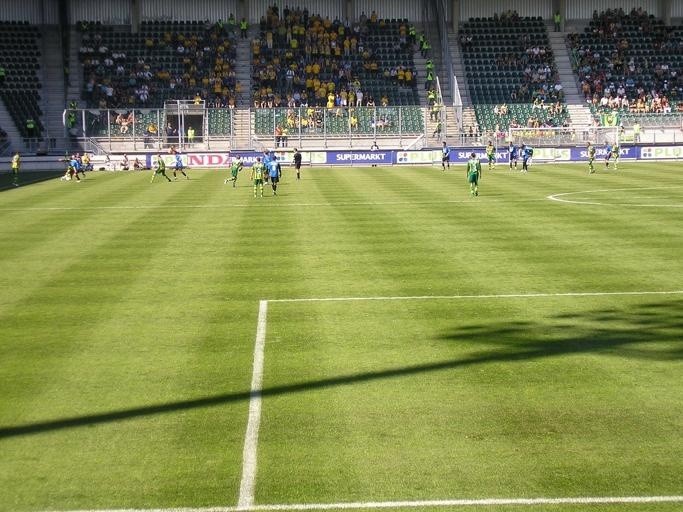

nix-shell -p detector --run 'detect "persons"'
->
[249,157,266,198]
[10,151,21,187]
[467,151,483,197]
[568,6,682,117]
[224,154,243,186]
[78,12,248,149]
[0,65,5,83]
[265,156,281,197]
[595,119,641,142]
[25,114,36,138]
[61,152,189,183]
[441,142,450,170]
[252,4,441,137]
[458,8,575,143]
[294,148,301,180]
[275,124,288,148]
[587,141,620,173]
[486,141,531,171]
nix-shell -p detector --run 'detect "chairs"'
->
[72,8,683,139]
[0,18,44,148]
